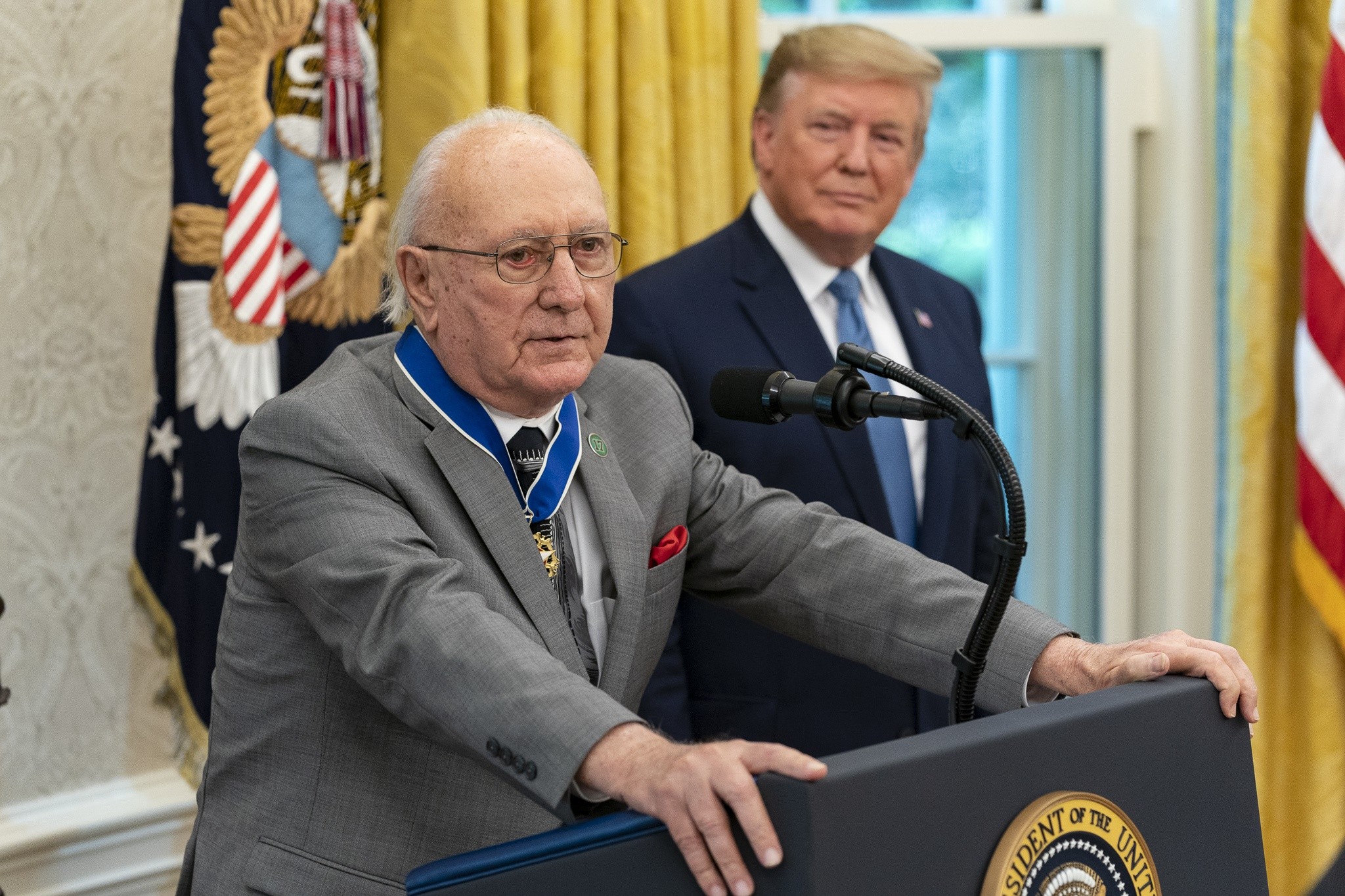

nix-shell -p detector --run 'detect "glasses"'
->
[417,230,628,284]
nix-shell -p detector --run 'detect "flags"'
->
[1291,2,1345,659]
[126,2,393,797]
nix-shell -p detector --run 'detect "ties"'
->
[828,269,919,550]
[505,426,600,688]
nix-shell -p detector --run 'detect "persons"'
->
[177,106,1260,896]
[602,28,1011,759]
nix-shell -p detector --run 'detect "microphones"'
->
[710,365,945,431]
